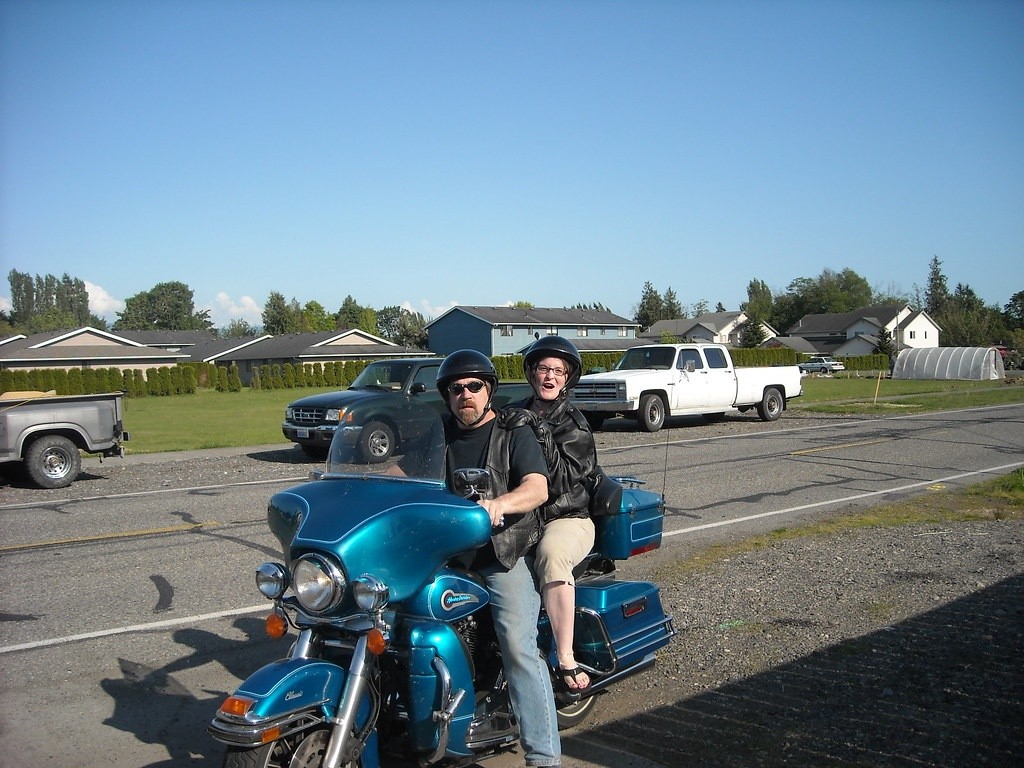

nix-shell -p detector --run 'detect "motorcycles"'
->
[207,393,679,768]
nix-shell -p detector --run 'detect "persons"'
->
[385,349,566,768]
[498,334,597,695]
[889,351,897,376]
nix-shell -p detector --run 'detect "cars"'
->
[795,356,846,374]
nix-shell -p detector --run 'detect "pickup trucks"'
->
[566,343,804,433]
[281,356,541,466]
[0,389,131,488]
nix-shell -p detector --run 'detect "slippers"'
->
[555,661,591,693]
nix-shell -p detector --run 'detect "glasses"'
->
[446,380,487,396]
[535,365,567,375]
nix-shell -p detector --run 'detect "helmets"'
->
[523,336,582,398]
[436,349,498,396]
